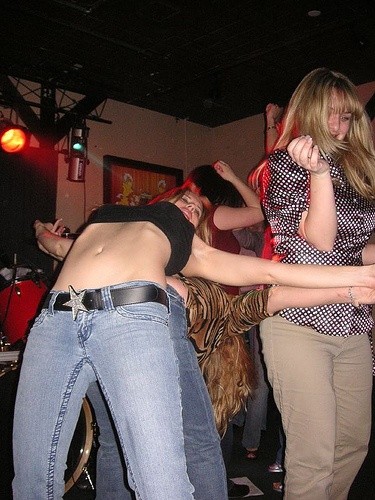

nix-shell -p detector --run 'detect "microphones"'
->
[66,151,86,183]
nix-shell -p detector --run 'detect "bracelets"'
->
[264,126,276,130]
[348,287,358,307]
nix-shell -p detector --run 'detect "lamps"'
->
[66,125,90,182]
[0,111,30,156]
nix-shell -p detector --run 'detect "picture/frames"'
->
[102,154,184,207]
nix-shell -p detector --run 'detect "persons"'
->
[11,188,375,500]
[34,96,375,499]
[81,274,375,500]
[257,67,375,500]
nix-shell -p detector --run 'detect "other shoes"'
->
[245,446,258,458]
[272,479,283,493]
[268,463,284,473]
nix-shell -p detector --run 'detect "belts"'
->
[42,284,169,313]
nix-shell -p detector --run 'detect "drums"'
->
[2,273,47,346]
[0,361,93,497]
